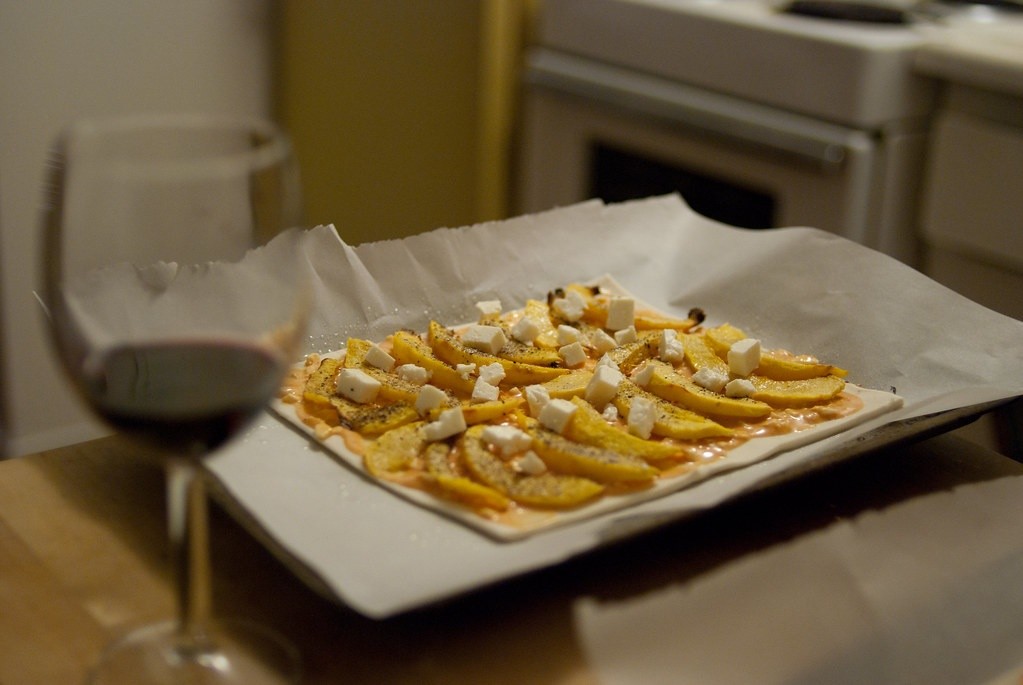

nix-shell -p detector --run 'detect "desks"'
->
[0,432,1023,685]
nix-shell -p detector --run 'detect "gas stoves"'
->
[532,0,1002,129]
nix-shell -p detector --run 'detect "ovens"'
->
[511,44,937,279]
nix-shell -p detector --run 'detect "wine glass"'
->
[41,114,310,685]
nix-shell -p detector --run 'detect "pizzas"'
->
[277,276,861,546]
